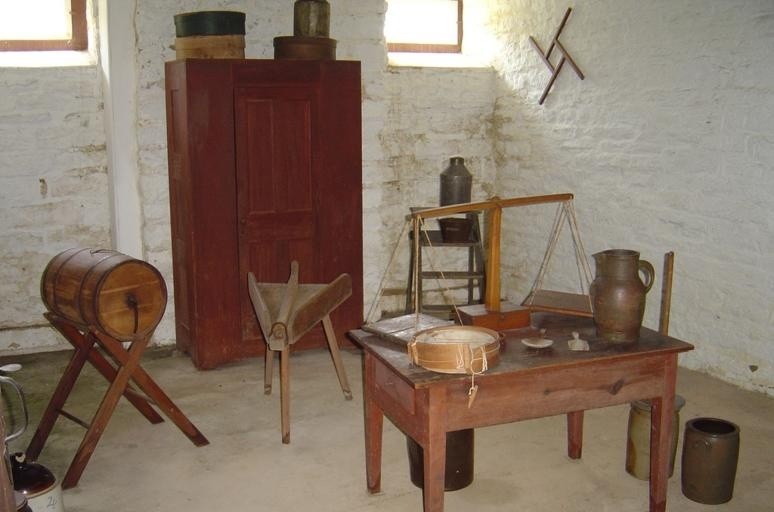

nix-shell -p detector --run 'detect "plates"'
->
[521,337,553,348]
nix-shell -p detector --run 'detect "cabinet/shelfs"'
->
[164,58,364,371]
[406,206,486,315]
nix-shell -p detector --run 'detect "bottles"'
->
[439,157,472,240]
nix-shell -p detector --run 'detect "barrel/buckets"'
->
[42,245,167,342]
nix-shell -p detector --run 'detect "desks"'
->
[345,311,695,512]
